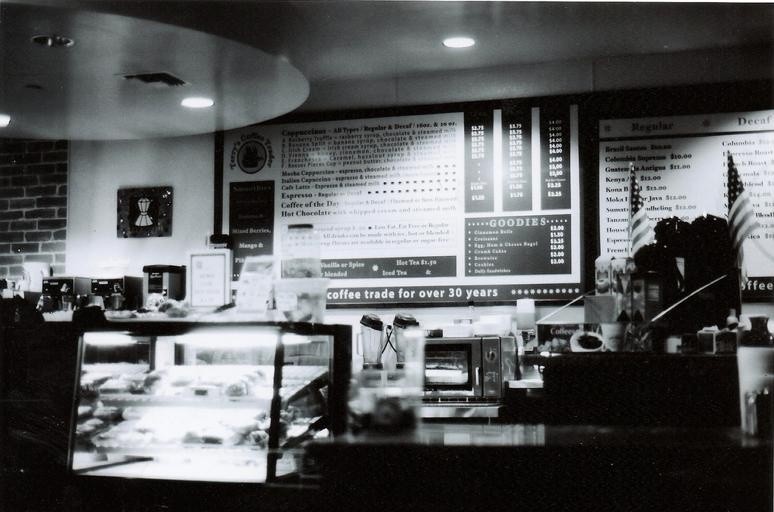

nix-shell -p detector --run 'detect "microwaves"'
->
[422,336,516,406]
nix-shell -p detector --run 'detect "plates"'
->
[569,332,602,352]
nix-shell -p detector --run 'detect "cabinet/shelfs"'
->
[61,318,352,510]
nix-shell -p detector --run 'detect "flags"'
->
[728,147,761,292]
[628,162,656,258]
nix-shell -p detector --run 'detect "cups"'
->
[599,323,624,351]
[516,298,536,330]
[424,315,513,339]
[354,314,382,370]
[270,276,331,323]
[385,313,419,368]
[666,338,681,353]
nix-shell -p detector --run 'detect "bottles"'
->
[733,314,773,437]
[280,223,325,277]
[727,308,738,332]
